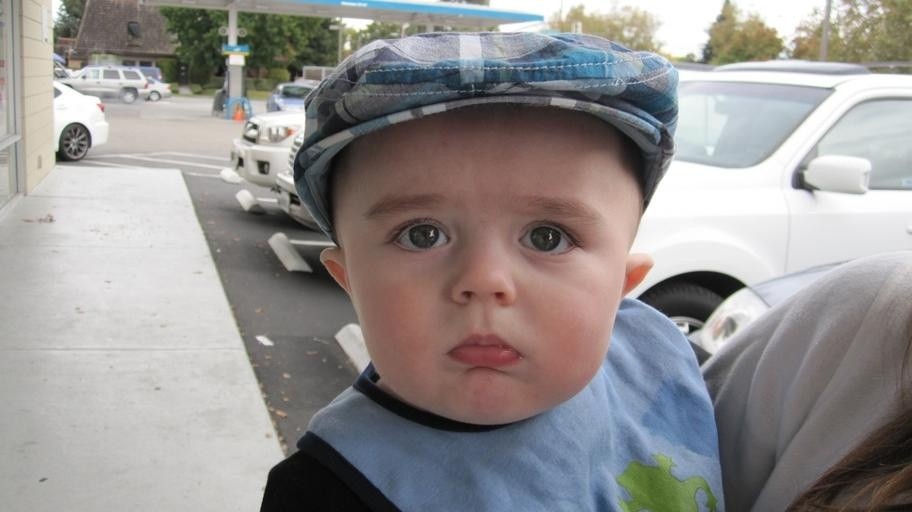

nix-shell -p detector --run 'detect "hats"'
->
[293,29,678,243]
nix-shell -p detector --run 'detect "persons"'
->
[702,234,912,512]
[259,31,725,512]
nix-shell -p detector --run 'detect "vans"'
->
[52,62,163,103]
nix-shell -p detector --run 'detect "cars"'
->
[53,79,110,160]
[141,76,172,103]
